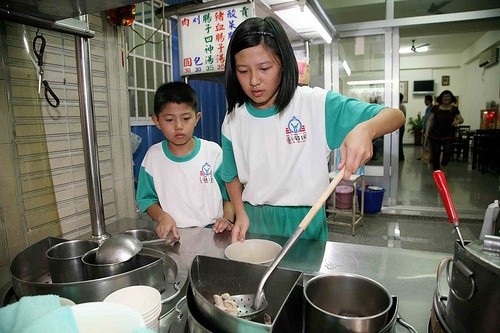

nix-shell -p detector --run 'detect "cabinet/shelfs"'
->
[480,109,498,129]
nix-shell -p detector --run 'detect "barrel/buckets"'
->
[446,241,500,333]
[335,186,353,209]
[357,185,385,215]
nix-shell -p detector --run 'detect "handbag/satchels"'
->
[421,139,430,164]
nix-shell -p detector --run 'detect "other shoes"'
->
[443,167,448,177]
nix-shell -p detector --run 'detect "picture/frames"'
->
[399,81,408,103]
[442,75,450,86]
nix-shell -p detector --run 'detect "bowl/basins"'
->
[82,247,137,279]
[102,286,163,333]
[57,297,75,308]
[304,274,392,333]
[225,239,283,264]
[45,240,100,283]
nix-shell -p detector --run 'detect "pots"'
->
[186,256,398,333]
[427,258,456,333]
[10,236,165,303]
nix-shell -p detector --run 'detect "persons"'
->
[222,18,405,244]
[369,90,464,174]
[136,81,234,239]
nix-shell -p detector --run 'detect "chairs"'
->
[456,125,470,158]
[324,148,365,237]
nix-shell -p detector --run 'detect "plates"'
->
[74,302,144,333]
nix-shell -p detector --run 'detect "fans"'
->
[411,40,430,53]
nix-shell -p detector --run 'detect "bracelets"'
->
[227,219,233,224]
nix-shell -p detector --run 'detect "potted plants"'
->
[406,115,425,146]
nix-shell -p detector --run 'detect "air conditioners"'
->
[478,47,500,68]
